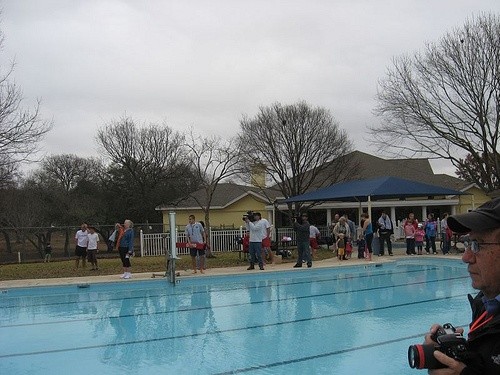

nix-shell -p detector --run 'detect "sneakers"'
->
[120,272,129,278]
[124,273,133,279]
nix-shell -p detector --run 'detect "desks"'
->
[438,233,466,255]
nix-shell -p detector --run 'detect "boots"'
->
[342,254,348,260]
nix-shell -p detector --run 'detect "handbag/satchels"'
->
[378,228,391,240]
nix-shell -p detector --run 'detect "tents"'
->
[276,176,475,203]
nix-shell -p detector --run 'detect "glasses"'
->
[464,240,500,254]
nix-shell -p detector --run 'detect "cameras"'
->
[408,329,467,369]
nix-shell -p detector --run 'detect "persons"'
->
[185,214,208,274]
[293,211,394,267]
[403,197,500,375]
[242,210,274,270]
[44,242,52,263]
[75,223,99,271]
[109,219,134,278]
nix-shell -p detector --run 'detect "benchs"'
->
[232,248,294,261]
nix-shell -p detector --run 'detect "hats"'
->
[447,198,500,234]
[338,216,346,222]
[418,223,422,228]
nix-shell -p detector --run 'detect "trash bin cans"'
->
[372,237,381,255]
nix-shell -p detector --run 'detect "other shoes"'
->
[260,265,265,270]
[307,262,312,267]
[378,253,381,256]
[433,252,437,255]
[427,252,430,255]
[411,253,414,256]
[389,252,393,256]
[405,252,408,256]
[246,265,254,270]
[293,262,302,267]
